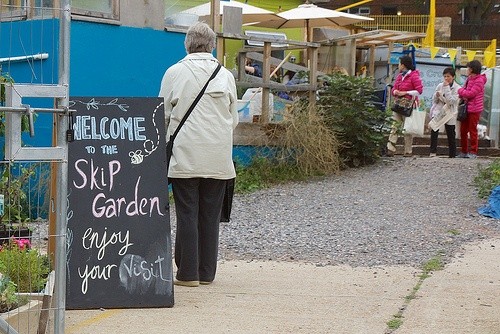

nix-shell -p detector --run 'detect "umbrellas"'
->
[165,0,288,67]
[243,0,375,67]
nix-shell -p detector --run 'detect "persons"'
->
[158,22,239,287]
[430,68,461,157]
[455,60,487,158]
[245,57,350,101]
[387,56,424,156]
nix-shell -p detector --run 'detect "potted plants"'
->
[0,276,43,334]
[0,165,37,244]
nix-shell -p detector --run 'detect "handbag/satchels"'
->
[457,100,467,121]
[166,140,173,185]
[391,96,413,117]
[402,101,426,137]
[220,162,236,223]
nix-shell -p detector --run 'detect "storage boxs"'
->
[237,100,254,124]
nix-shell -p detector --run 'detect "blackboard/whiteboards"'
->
[65,95,175,311]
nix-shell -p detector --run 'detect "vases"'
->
[14,270,54,334]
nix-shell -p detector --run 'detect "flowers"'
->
[0,236,51,293]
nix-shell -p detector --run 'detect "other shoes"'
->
[383,151,394,157]
[429,152,436,157]
[174,278,210,287]
[454,153,477,158]
[403,153,412,157]
